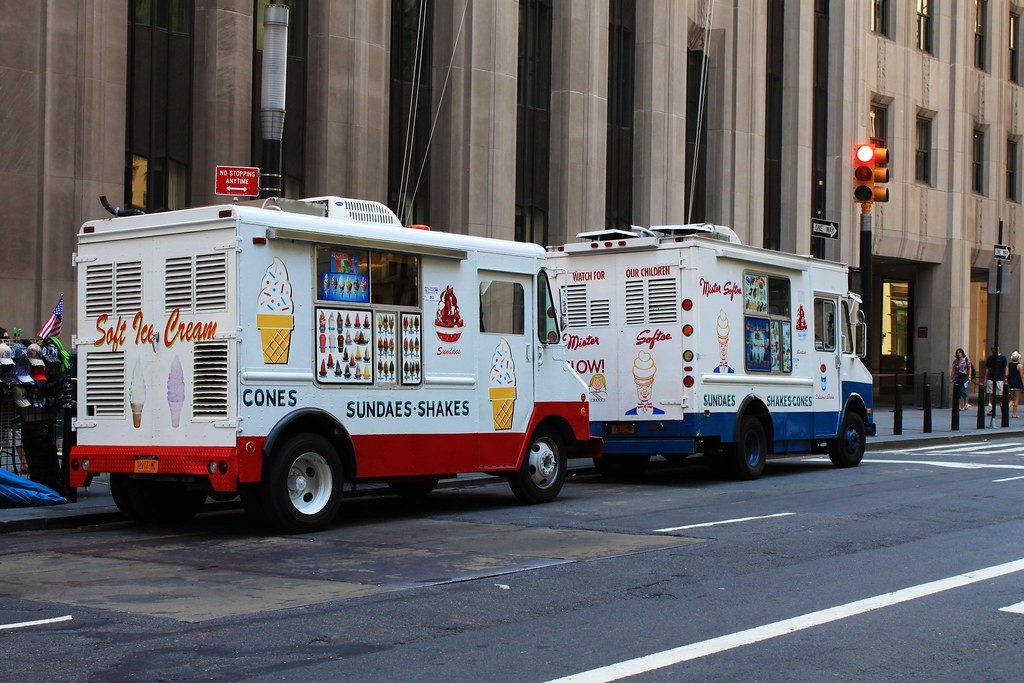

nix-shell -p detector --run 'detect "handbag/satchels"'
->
[966,358,976,380]
[957,372,967,385]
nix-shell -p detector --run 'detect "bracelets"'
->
[969,377,971,378]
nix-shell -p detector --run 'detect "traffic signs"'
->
[214,166,260,197]
[992,243,1012,262]
[810,217,839,240]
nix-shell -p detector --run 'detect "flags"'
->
[35,293,63,340]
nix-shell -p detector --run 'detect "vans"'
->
[544,223,877,483]
[69,195,603,535]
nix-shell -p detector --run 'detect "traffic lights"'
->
[852,144,875,201]
[874,146,890,202]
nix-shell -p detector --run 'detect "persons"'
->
[983,345,1008,416]
[950,348,972,412]
[0,328,30,473]
[1005,350,1024,418]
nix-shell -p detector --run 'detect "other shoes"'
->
[1012,415,1020,418]
[21,467,29,474]
[964,404,972,410]
[987,410,992,415]
[959,407,965,411]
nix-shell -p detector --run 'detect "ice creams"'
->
[324,274,367,299]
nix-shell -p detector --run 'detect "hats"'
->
[0,341,72,409]
[1011,351,1021,360]
